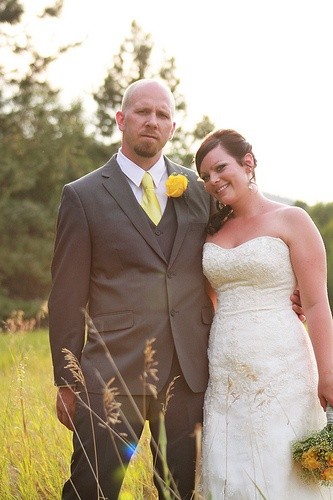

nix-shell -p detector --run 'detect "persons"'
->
[194,129,333,500]
[48,78,308,500]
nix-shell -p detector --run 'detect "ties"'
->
[140,172,161,226]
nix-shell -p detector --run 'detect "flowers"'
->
[292,403,333,489]
[164,172,201,221]
